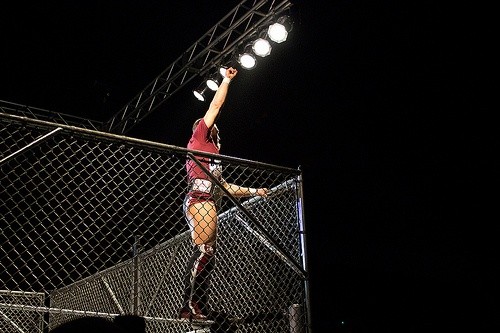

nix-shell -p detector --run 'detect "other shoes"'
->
[178,311,224,323]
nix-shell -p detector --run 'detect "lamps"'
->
[191,13,295,103]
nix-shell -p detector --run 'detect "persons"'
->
[182,66,268,321]
